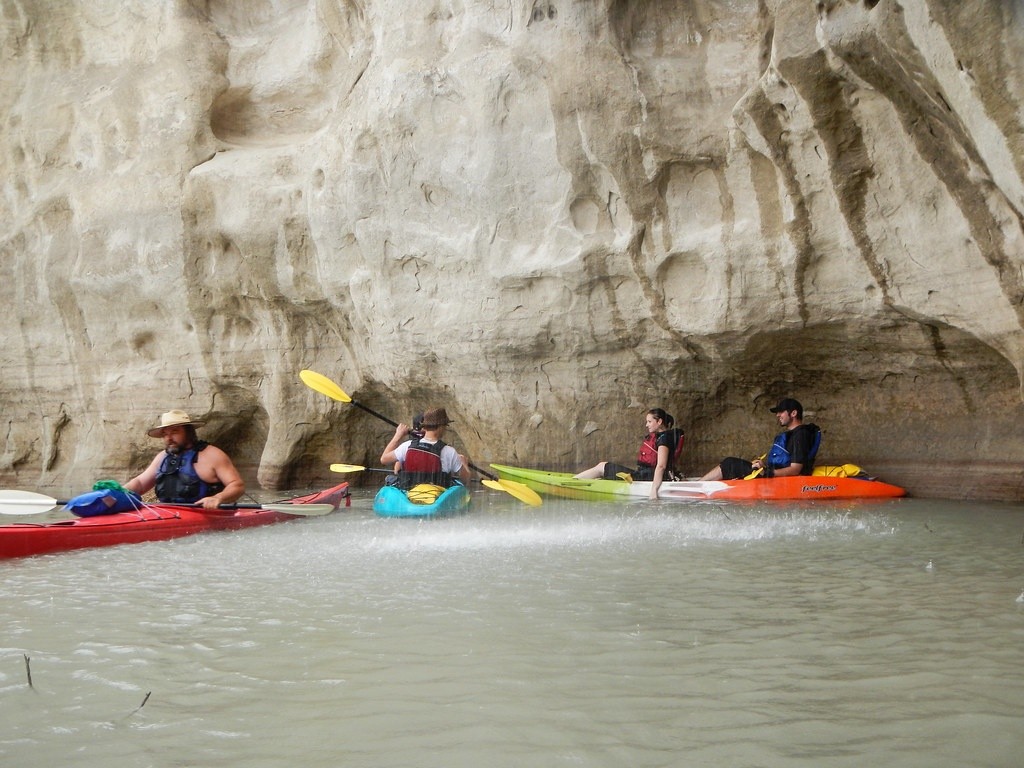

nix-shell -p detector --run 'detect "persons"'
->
[123,410,246,509]
[381,407,471,485]
[668,398,812,482]
[572,408,674,500]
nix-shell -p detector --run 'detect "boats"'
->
[489,459,907,501]
[368,478,474,521]
[0,479,351,560]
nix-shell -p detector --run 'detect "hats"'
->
[413,415,424,432]
[770,399,803,415]
[419,408,454,426]
[148,411,207,438]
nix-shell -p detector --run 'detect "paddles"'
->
[616,470,636,486]
[744,452,768,481]
[330,462,505,492]
[0,489,337,518]
[298,368,543,508]
